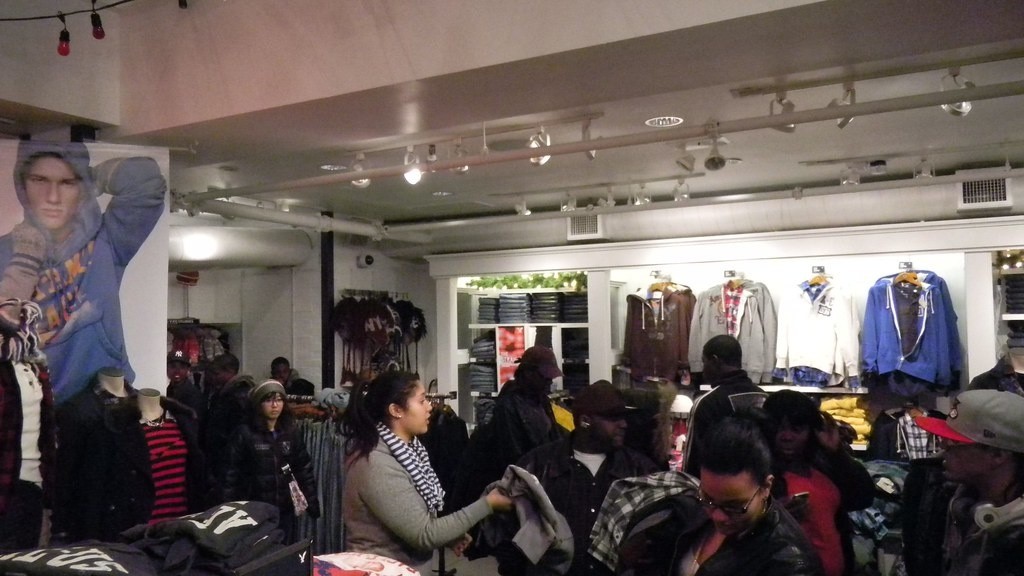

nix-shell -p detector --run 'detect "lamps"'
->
[769,80,854,132]
[677,126,725,171]
[349,153,371,188]
[525,114,602,166]
[941,69,977,117]
[791,153,943,198]
[517,177,690,216]
[403,121,490,184]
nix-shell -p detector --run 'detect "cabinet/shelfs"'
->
[467,287,589,397]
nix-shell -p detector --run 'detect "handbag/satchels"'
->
[285,472,309,517]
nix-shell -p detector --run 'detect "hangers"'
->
[894,263,921,287]
[807,266,827,286]
[168,318,202,333]
[549,389,573,407]
[646,271,675,302]
[722,270,746,290]
[425,392,454,418]
[286,395,337,423]
[672,410,691,438]
[903,398,924,420]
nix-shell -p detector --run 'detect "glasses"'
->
[936,437,985,455]
[694,477,765,519]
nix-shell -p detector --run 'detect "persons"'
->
[0,141,168,399]
[0,296,187,552]
[339,370,514,576]
[964,346,1024,399]
[483,336,1024,576]
[167,347,321,547]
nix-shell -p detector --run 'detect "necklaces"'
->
[689,533,707,576]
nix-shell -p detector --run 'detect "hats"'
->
[515,345,568,379]
[915,388,1024,453]
[247,379,287,412]
[572,380,645,418]
[168,349,191,368]
